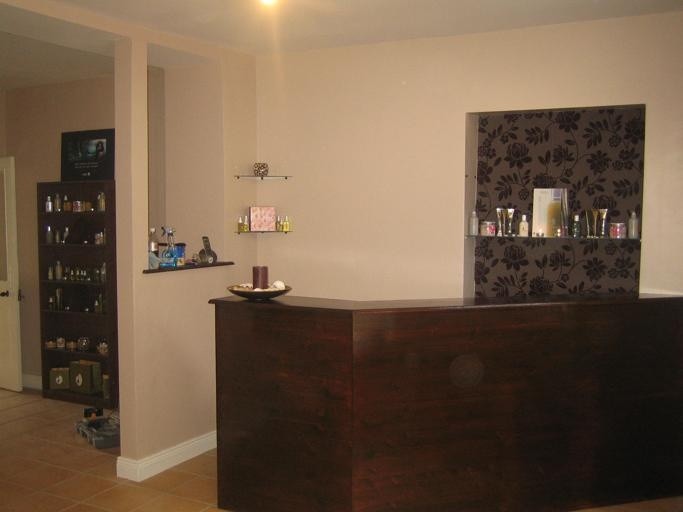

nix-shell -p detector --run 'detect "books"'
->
[48,366,69,389]
[248,205,276,231]
[100,372,112,401]
[67,363,93,396]
[73,359,104,393]
[531,186,568,239]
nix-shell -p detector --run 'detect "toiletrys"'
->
[516,214,528,237]
[570,213,581,239]
[276,216,280,230]
[243,215,249,232]
[237,216,243,232]
[42,190,108,354]
[279,217,282,231]
[282,215,289,231]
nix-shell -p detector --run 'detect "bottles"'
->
[277,215,290,231]
[237,215,249,232]
[518,214,530,237]
[55,260,63,280]
[148,227,159,257]
[572,214,582,236]
[63,226,70,241]
[45,224,54,243]
[45,193,71,213]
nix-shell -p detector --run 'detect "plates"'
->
[227,283,292,301]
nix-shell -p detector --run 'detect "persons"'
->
[94,142,105,166]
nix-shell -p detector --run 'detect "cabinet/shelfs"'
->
[236,172,292,233]
[36,180,118,411]
